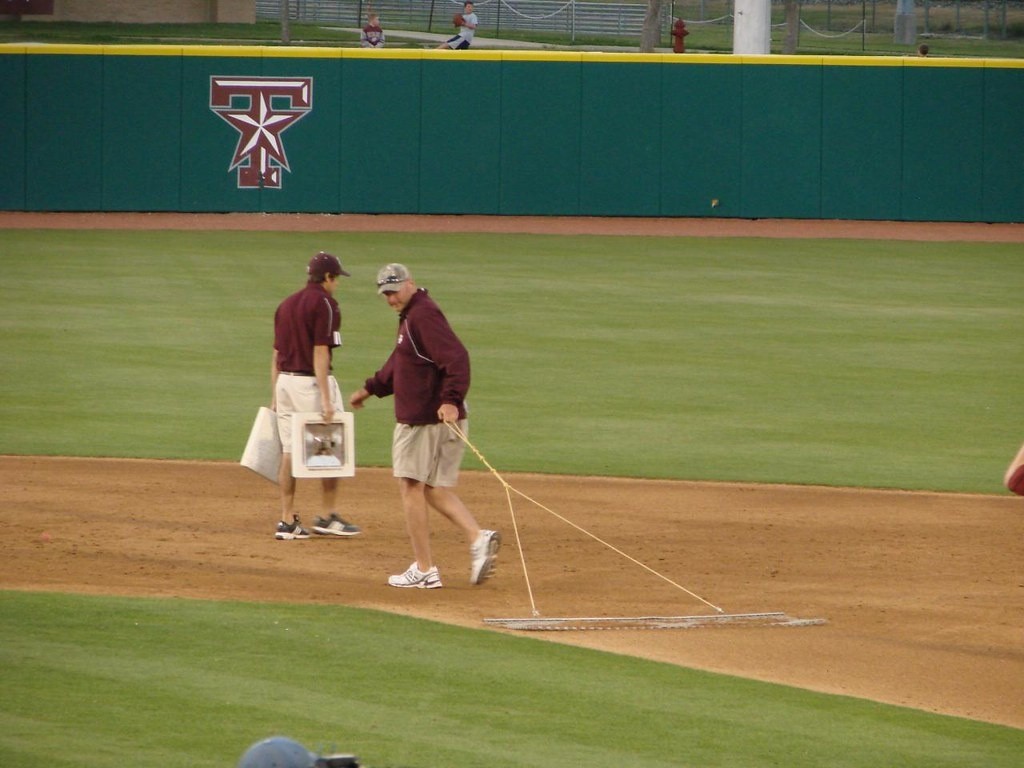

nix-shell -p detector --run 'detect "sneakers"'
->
[388,561,443,589]
[469,529,501,585]
[275,515,310,540]
[311,514,361,535]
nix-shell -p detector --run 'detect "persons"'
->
[1004,444,1024,496]
[361,13,385,48]
[918,45,929,57]
[307,439,340,466]
[351,263,499,588]
[436,2,478,50]
[272,254,362,539]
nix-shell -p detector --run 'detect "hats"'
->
[308,253,350,276]
[376,263,408,295]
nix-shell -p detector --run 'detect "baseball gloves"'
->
[454,14,465,26]
